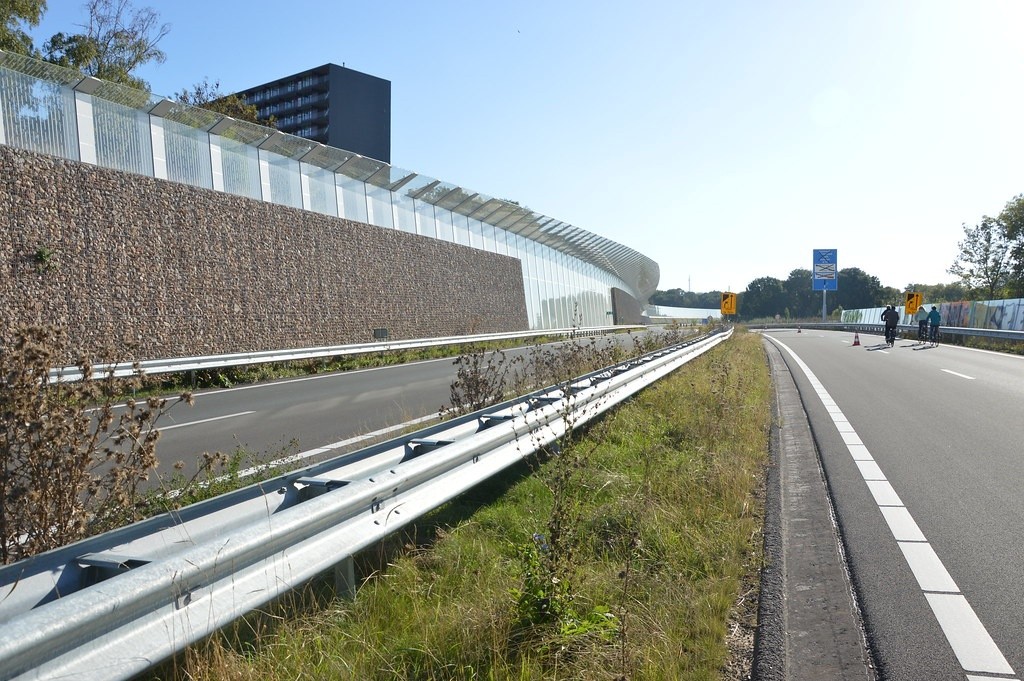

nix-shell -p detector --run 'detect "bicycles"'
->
[918,325,928,344]
[929,326,940,347]
[882,320,896,347]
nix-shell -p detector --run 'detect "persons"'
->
[926,306,942,340]
[881,305,900,341]
[915,306,929,340]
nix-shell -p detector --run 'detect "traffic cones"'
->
[797,323,801,334]
[852,331,861,346]
